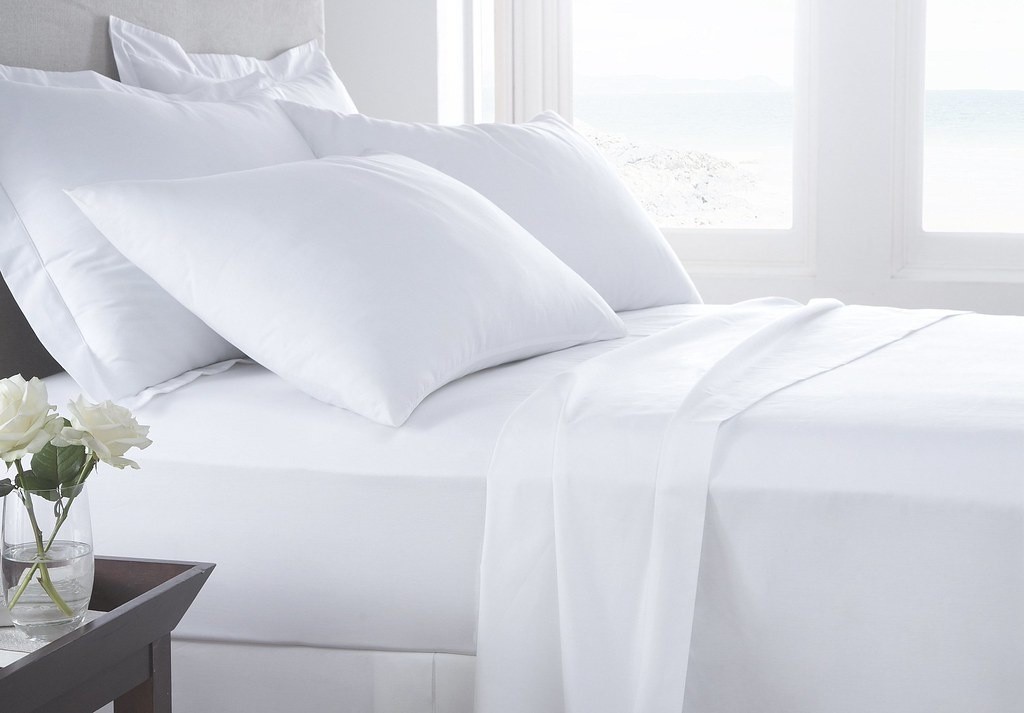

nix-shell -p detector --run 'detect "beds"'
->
[2,287,1024,713]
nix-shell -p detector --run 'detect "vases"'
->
[0,481,96,639]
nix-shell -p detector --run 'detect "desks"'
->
[0,540,218,713]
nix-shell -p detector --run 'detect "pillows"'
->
[107,13,360,124]
[60,145,632,433]
[0,64,328,419]
[279,95,709,310]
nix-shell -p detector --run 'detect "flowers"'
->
[0,372,155,620]
